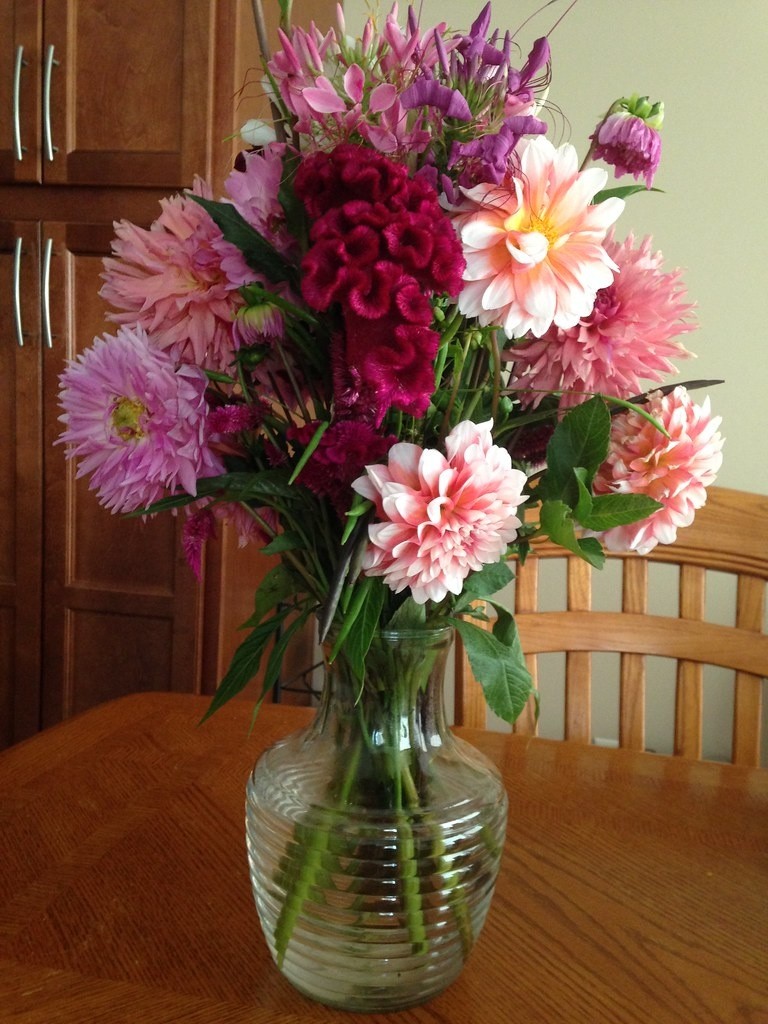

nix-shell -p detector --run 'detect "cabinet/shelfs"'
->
[0,3,343,743]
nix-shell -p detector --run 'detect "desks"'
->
[0,690,768,1024]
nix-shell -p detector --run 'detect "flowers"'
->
[55,0,724,633]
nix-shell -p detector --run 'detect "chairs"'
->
[439,475,768,768]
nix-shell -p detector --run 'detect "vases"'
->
[243,606,509,1012]
